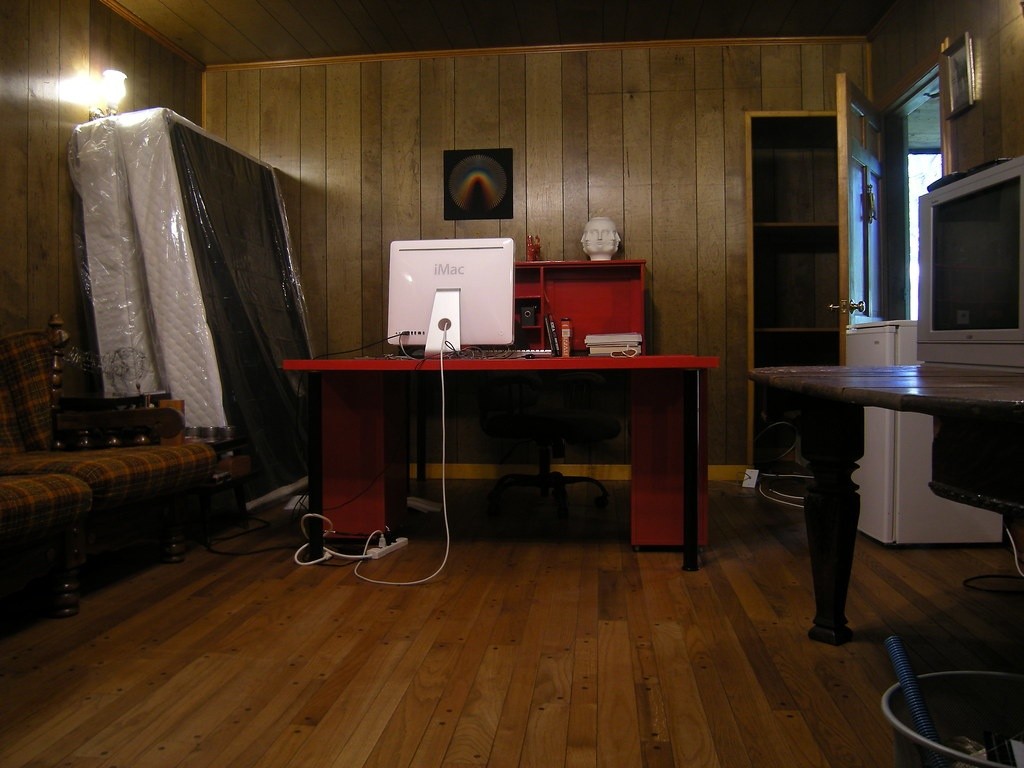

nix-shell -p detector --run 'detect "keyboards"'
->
[466,349,554,358]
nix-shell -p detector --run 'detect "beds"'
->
[75,116,167,396]
[121,108,312,508]
[89,70,127,123]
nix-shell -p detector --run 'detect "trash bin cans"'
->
[881,671,1024,768]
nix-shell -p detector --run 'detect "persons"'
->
[580,217,621,262]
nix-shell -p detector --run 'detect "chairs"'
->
[0,329,215,562]
[0,370,93,616]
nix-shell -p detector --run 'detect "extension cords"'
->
[368,537,408,559]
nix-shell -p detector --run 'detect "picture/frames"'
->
[940,30,978,120]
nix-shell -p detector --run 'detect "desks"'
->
[751,366,1024,646]
[283,355,721,552]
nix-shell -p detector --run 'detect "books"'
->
[583,332,643,357]
[545,312,571,357]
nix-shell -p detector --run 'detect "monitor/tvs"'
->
[389,237,515,357]
[916,155,1024,368]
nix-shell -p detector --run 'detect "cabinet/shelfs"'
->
[515,260,645,355]
[745,110,837,485]
